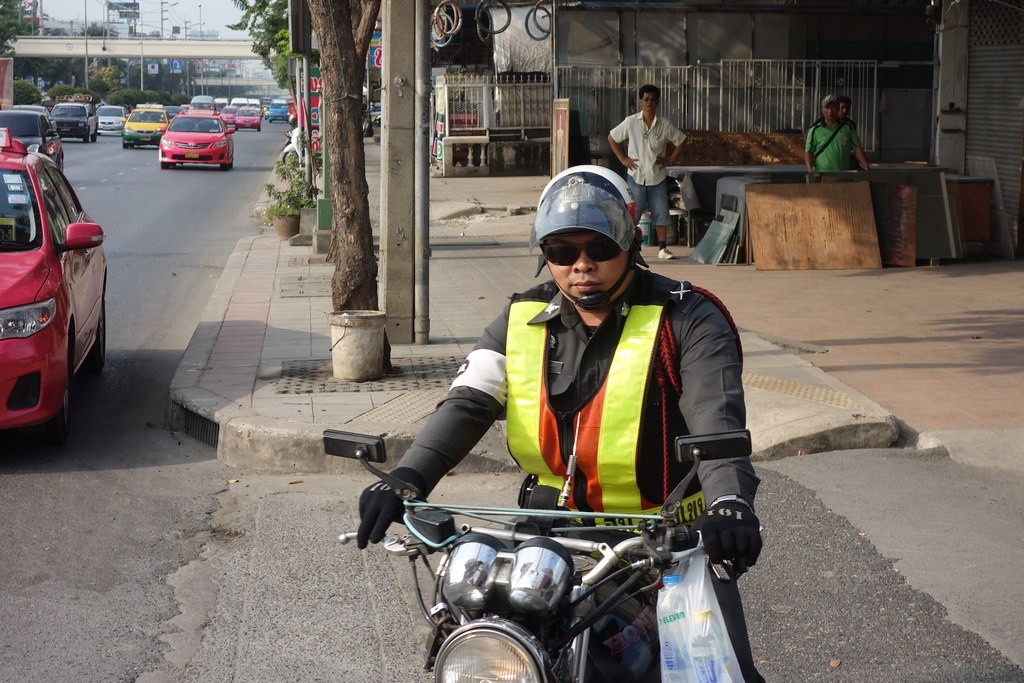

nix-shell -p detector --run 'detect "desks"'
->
[664,165,960,268]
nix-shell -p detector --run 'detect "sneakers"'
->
[658,248,675,260]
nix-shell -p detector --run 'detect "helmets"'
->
[529,164,649,278]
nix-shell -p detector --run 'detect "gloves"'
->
[357,467,427,550]
[689,500,763,573]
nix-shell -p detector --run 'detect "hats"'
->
[822,93,837,107]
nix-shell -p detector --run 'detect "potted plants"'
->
[264,133,324,237]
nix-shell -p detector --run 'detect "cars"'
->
[163,95,229,122]
[159,112,237,171]
[215,96,289,132]
[0,94,99,172]
[0,126,107,443]
[96,104,169,149]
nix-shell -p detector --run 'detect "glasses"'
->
[640,97,657,102]
[539,238,621,266]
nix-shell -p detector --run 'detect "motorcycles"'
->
[323,428,757,683]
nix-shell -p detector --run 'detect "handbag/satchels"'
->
[658,550,741,682]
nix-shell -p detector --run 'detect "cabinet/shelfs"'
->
[944,175,996,245]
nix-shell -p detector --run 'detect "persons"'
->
[356,165,763,683]
[805,95,869,183]
[814,96,855,130]
[608,84,687,259]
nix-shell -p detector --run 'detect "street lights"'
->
[187,4,206,103]
[103,0,180,93]
